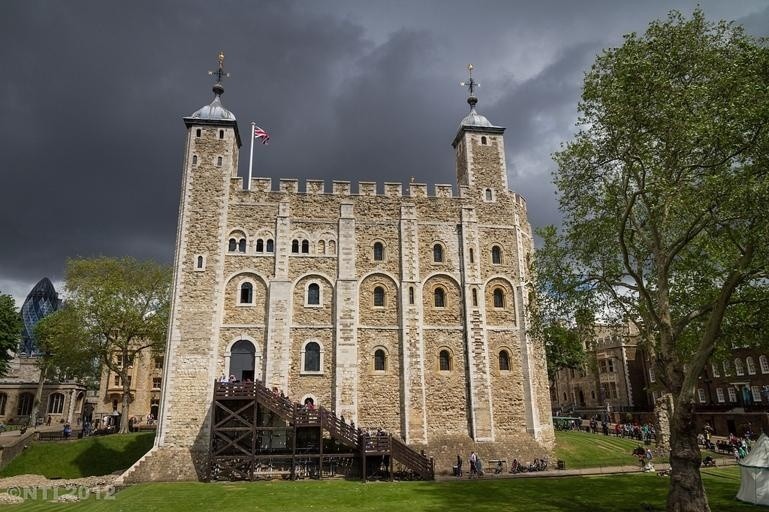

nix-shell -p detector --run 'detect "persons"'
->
[558,415,756,476]
[46,415,51,425]
[20,422,27,433]
[453,454,464,477]
[420,450,428,471]
[218,373,390,450]
[64,414,155,439]
[468,450,547,475]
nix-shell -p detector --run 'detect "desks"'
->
[488,459,507,472]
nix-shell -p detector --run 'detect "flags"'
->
[254,125,270,145]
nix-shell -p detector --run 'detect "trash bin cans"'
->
[558,461,565,470]
[453,467,458,476]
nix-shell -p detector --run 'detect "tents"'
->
[736,431,769,506]
